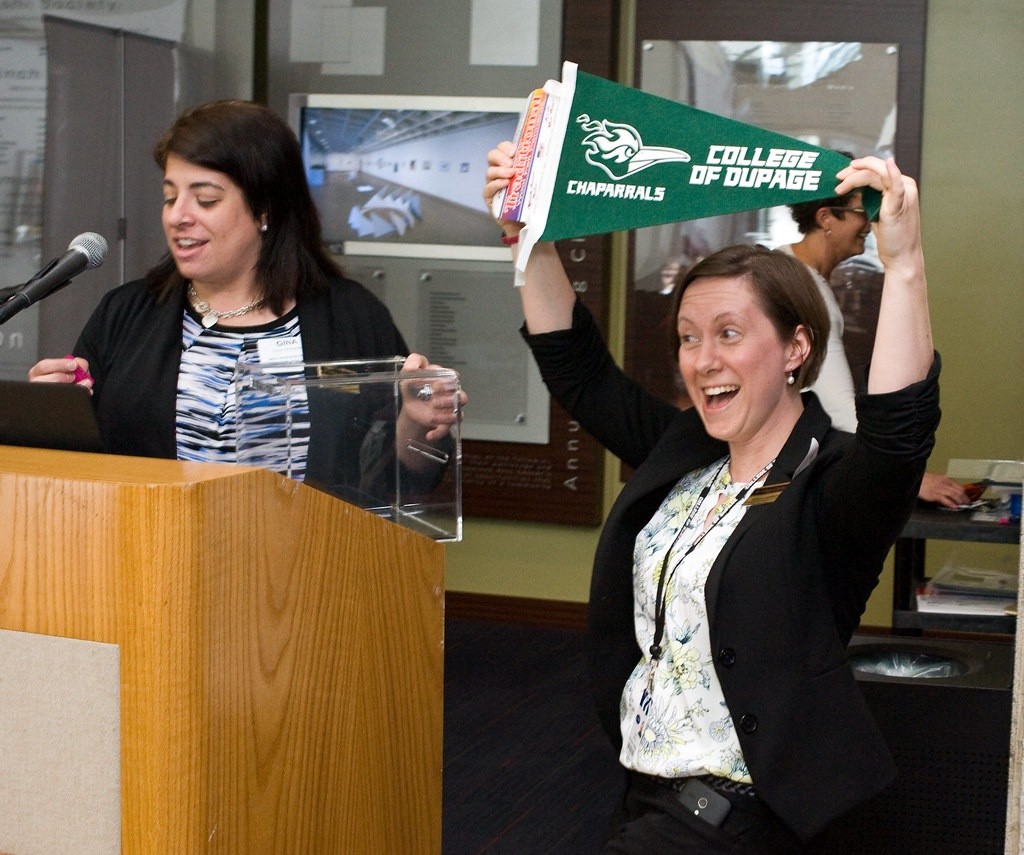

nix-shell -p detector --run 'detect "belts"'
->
[647,773,758,794]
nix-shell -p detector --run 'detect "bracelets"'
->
[499,229,518,248]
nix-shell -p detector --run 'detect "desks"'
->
[892,498,1022,637]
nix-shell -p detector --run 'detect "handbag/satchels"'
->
[341,278,396,514]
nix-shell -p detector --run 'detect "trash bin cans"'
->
[822,627,1006,855]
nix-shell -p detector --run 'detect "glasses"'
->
[828,206,880,223]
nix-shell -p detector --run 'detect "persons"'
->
[774,148,969,509]
[25,97,468,516]
[484,141,942,855]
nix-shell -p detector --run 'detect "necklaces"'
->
[188,280,266,328]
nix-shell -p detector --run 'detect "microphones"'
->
[0,231,109,326]
